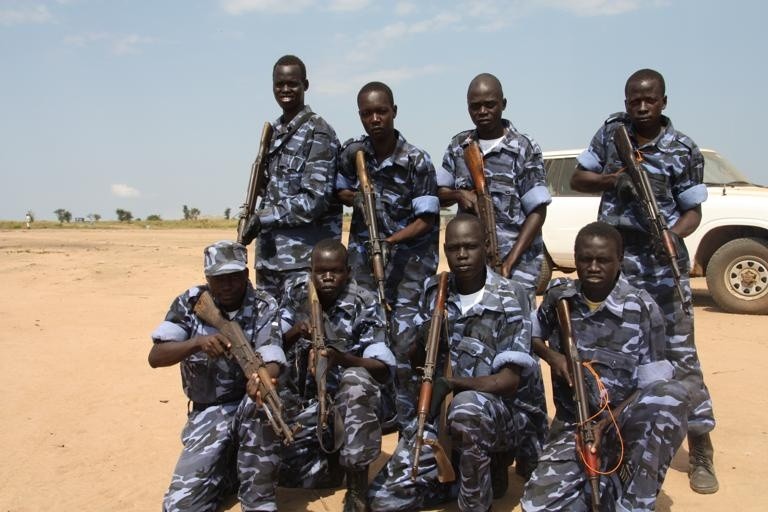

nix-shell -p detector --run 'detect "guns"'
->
[352,148,394,310]
[553,298,625,511]
[406,270,460,485]
[609,126,684,279]
[303,277,346,453]
[461,142,510,278]
[235,122,273,241]
[191,290,307,447]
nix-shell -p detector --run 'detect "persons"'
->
[336,81,440,438]
[570,68,720,494]
[370,214,532,506]
[278,237,398,512]
[25,214,31,230]
[147,240,279,512]
[236,56,341,330]
[439,74,553,437]
[521,223,689,512]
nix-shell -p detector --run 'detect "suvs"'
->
[537,147,768,316]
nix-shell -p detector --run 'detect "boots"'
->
[342,466,369,512]
[686,431,719,494]
[491,463,509,502]
[513,455,536,478]
